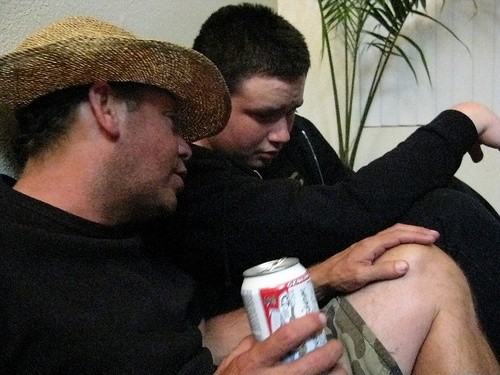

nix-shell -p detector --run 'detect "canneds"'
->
[240,257,327,364]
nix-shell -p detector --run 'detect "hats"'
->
[0,15,233,144]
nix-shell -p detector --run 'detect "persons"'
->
[0,15,499,375]
[139,3,500,362]
